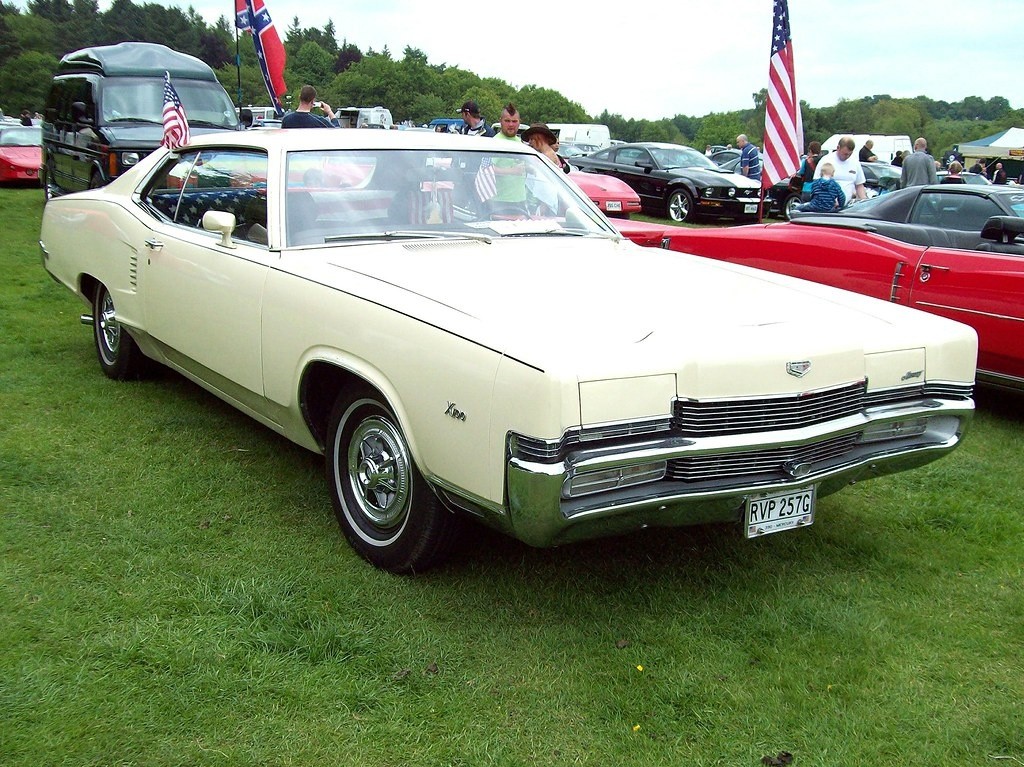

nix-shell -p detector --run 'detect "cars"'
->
[0,116,43,187]
[491,140,1024,383]
[252,119,282,127]
[39,128,979,576]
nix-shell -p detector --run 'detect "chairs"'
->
[246,191,318,231]
[387,182,454,224]
[975,216,1024,255]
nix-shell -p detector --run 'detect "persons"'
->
[450,100,496,210]
[20,109,44,126]
[489,102,528,214]
[281,84,341,128]
[521,122,571,219]
[703,132,1024,213]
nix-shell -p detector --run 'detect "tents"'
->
[957,127,1024,158]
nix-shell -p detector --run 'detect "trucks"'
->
[235,104,394,128]
[491,123,611,155]
[427,118,465,134]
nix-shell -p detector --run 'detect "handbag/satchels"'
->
[788,173,805,194]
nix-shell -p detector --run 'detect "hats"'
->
[456,102,481,114]
[521,123,556,145]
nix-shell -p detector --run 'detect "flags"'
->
[160,68,191,150]
[474,157,498,201]
[235,0,288,117]
[762,0,804,189]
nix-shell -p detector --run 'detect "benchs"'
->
[150,189,408,243]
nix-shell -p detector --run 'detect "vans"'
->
[821,134,914,165]
[39,43,254,200]
